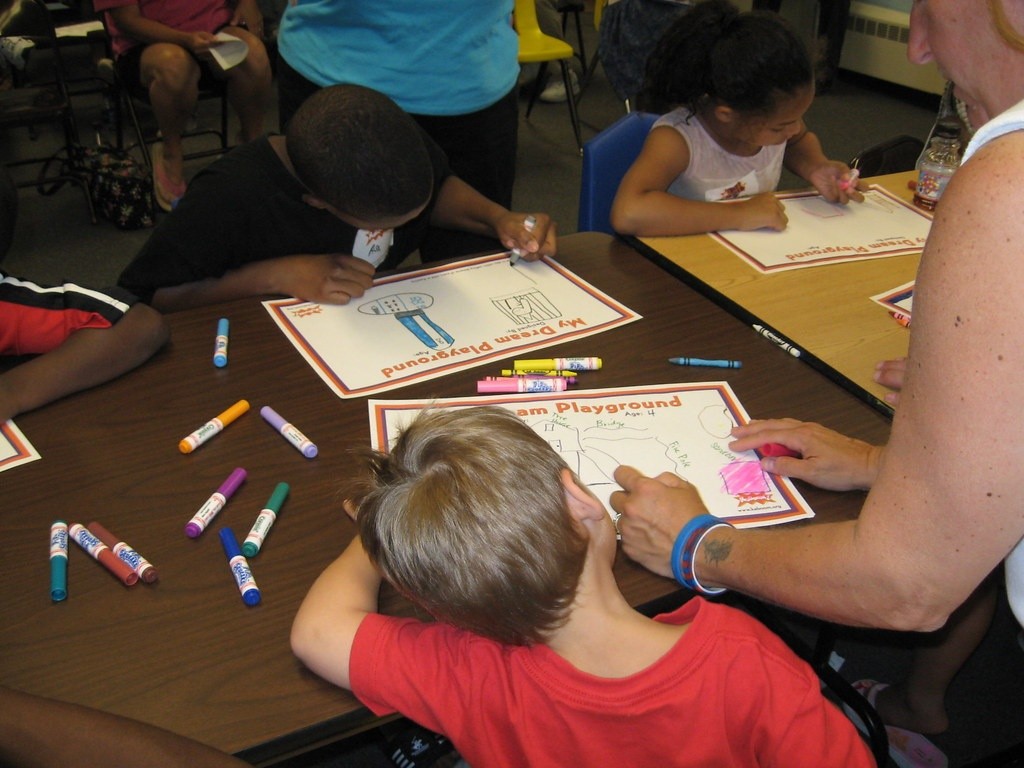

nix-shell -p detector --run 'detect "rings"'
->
[612,513,622,534]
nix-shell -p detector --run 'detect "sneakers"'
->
[540,70,580,102]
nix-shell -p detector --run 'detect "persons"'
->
[0,268,170,427]
[118,86,557,311]
[0,688,254,768]
[92,0,272,210]
[276,0,579,263]
[290,404,877,768]
[610,0,1024,768]
[609,0,869,237]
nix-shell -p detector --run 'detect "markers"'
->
[48,519,159,600]
[213,317,229,368]
[477,377,568,393]
[260,406,318,458]
[514,356,602,371]
[840,166,859,192]
[176,399,252,453]
[186,468,291,608]
[508,213,537,267]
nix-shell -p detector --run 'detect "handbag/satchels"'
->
[37,143,154,230]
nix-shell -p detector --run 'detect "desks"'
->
[0,231,894,768]
[615,166,936,422]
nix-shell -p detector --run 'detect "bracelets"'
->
[671,515,727,590]
[682,521,737,596]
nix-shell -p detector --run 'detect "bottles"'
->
[913,120,963,212]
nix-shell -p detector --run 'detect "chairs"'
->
[580,112,664,232]
[512,0,697,160]
[850,136,926,178]
[0,1,280,225]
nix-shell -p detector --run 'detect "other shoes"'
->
[148,144,187,211]
